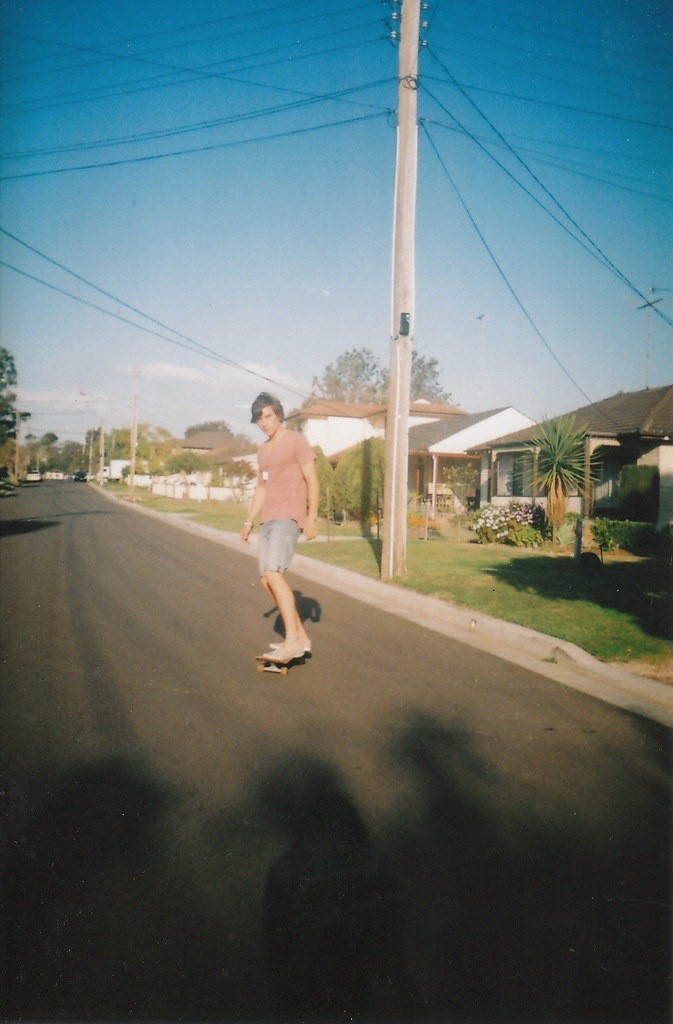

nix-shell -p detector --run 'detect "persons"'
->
[238,391,319,660]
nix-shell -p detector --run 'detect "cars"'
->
[25,469,42,482]
[73,471,87,482]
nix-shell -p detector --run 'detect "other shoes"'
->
[269,638,312,652]
[263,646,305,663]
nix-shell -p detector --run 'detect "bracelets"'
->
[244,522,253,528]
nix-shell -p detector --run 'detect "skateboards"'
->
[254,655,293,677]
